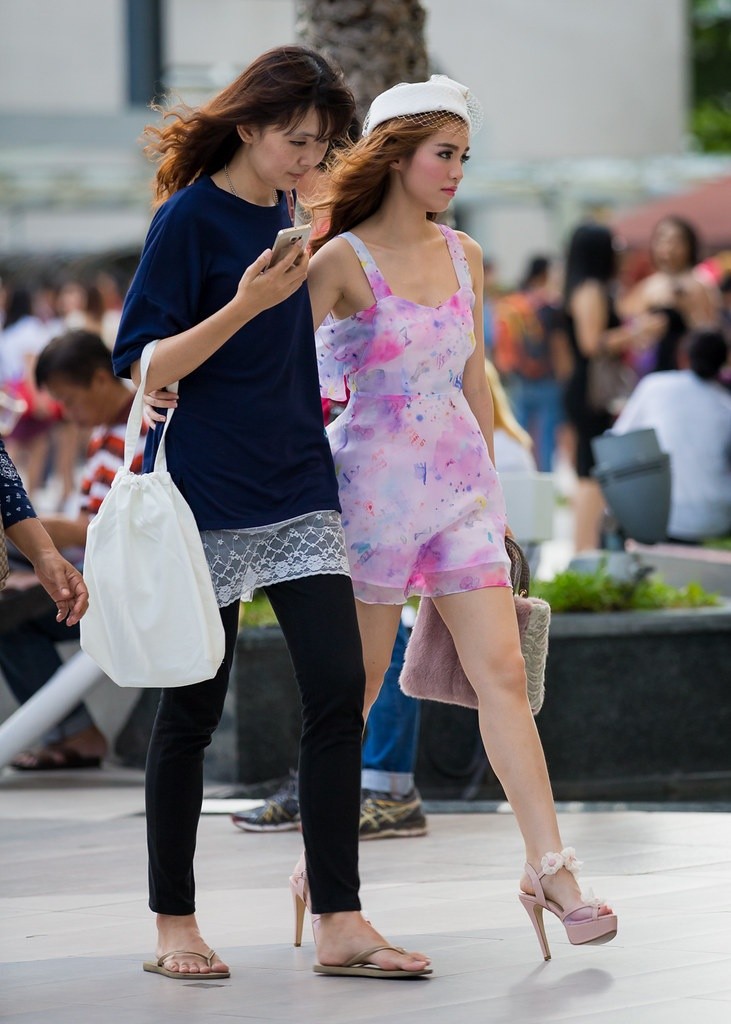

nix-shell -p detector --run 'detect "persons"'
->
[141,73,618,962]
[111,42,436,981]
[0,214,731,844]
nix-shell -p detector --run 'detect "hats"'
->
[361,74,481,132]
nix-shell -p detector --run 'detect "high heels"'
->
[518,847,618,960]
[287,853,313,947]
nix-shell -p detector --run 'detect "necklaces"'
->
[222,160,279,207]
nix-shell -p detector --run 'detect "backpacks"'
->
[494,293,550,381]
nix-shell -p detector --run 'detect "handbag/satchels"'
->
[78,338,226,687]
[588,332,633,409]
[397,538,551,716]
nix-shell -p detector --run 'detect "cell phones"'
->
[265,223,311,271]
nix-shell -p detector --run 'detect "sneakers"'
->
[356,787,430,839]
[230,787,305,831]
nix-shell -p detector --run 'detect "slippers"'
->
[143,950,231,979]
[312,947,433,977]
[8,741,103,771]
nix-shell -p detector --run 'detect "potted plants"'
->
[229,551,731,801]
